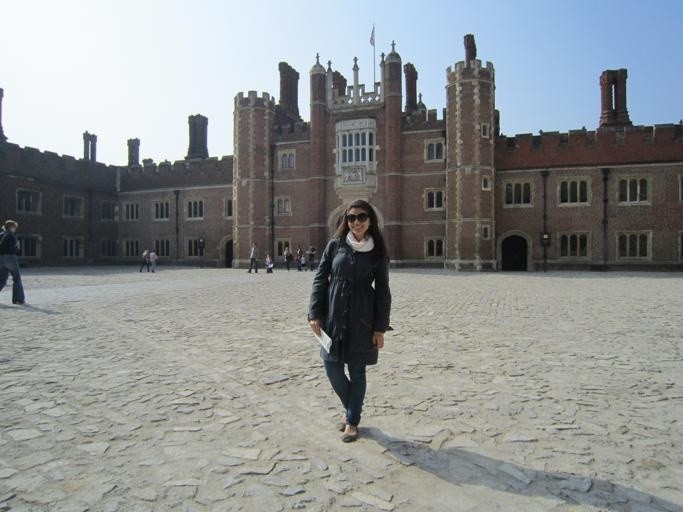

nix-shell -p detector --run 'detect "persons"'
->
[303,198,394,444]
[294,244,302,272]
[282,245,291,272]
[0,219,27,305]
[246,241,261,274]
[263,253,274,274]
[149,249,158,273]
[307,244,316,272]
[138,247,150,272]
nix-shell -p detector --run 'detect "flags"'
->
[368,26,375,48]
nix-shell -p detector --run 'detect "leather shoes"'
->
[339,422,347,432]
[341,429,359,442]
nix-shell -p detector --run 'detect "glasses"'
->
[346,213,371,223]
